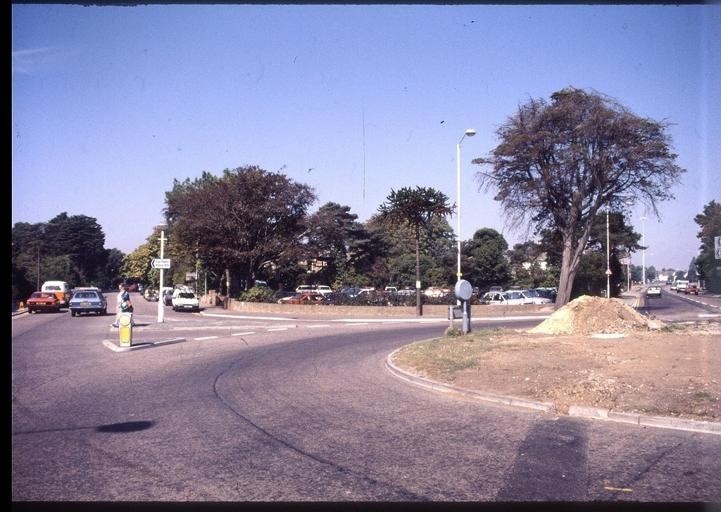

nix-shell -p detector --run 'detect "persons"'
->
[111,283,136,328]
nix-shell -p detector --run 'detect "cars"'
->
[137,282,199,312]
[646,286,662,299]
[26,280,107,317]
[670,280,700,295]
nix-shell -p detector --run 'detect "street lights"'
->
[640,217,647,284]
[456,129,475,307]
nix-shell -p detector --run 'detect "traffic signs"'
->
[151,258,171,269]
